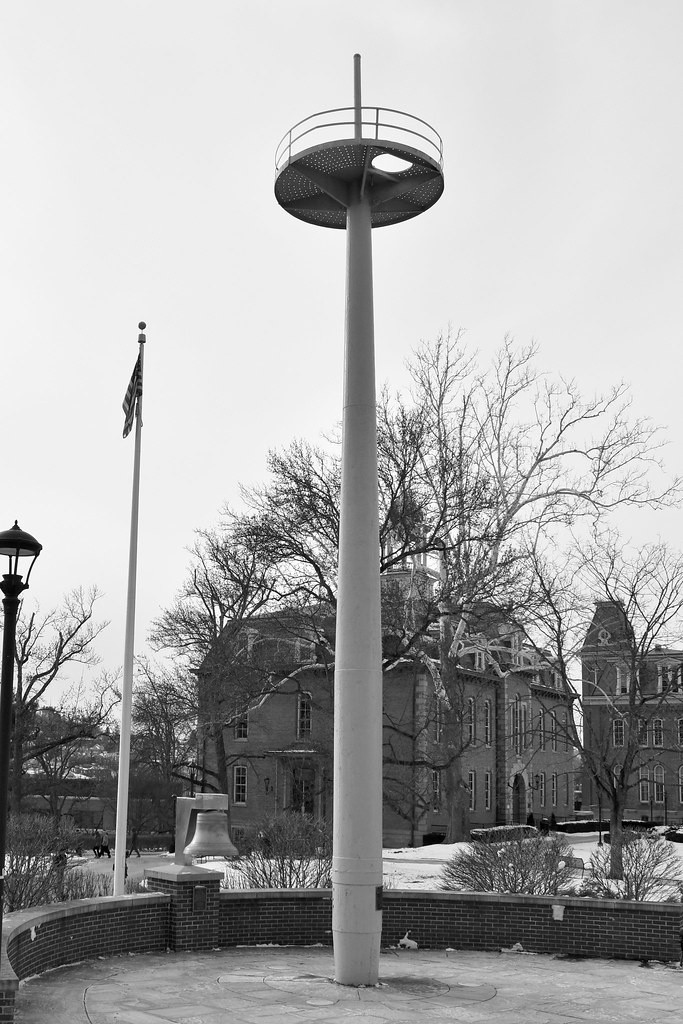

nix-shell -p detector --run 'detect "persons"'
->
[76,845,83,857]
[99,832,111,858]
[129,827,140,857]
[93,831,100,858]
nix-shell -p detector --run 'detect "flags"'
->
[122,349,143,439]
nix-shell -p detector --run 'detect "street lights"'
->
[0,520,43,941]
[186,760,197,797]
[171,793,178,852]
[649,795,654,821]
[596,791,603,846]
